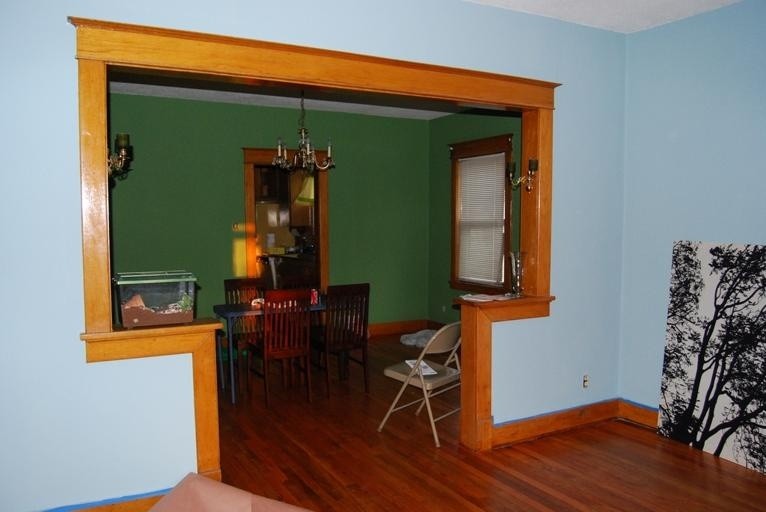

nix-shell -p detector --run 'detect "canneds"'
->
[311,289,319,305]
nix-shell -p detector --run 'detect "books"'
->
[405,360,437,375]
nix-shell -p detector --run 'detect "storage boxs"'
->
[113,266,200,332]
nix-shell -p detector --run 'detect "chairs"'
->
[309,283,372,400]
[373,317,464,449]
[238,287,318,411]
[221,276,286,376]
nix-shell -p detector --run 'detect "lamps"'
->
[105,130,134,180]
[504,157,541,196]
[271,90,336,176]
